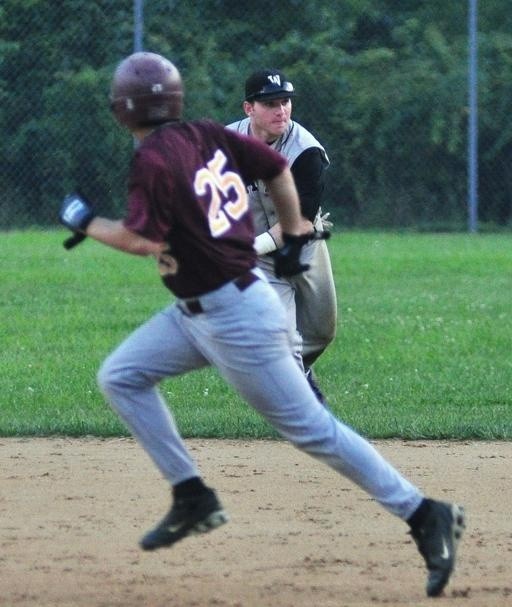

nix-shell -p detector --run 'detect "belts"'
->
[177,272,259,318]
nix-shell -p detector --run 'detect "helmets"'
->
[111,52,182,126]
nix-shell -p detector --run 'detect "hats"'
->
[246,69,297,101]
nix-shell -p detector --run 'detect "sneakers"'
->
[142,491,226,549]
[408,500,464,598]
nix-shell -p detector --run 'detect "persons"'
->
[218,68,338,412]
[56,51,466,601]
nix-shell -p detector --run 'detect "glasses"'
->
[248,82,294,98]
